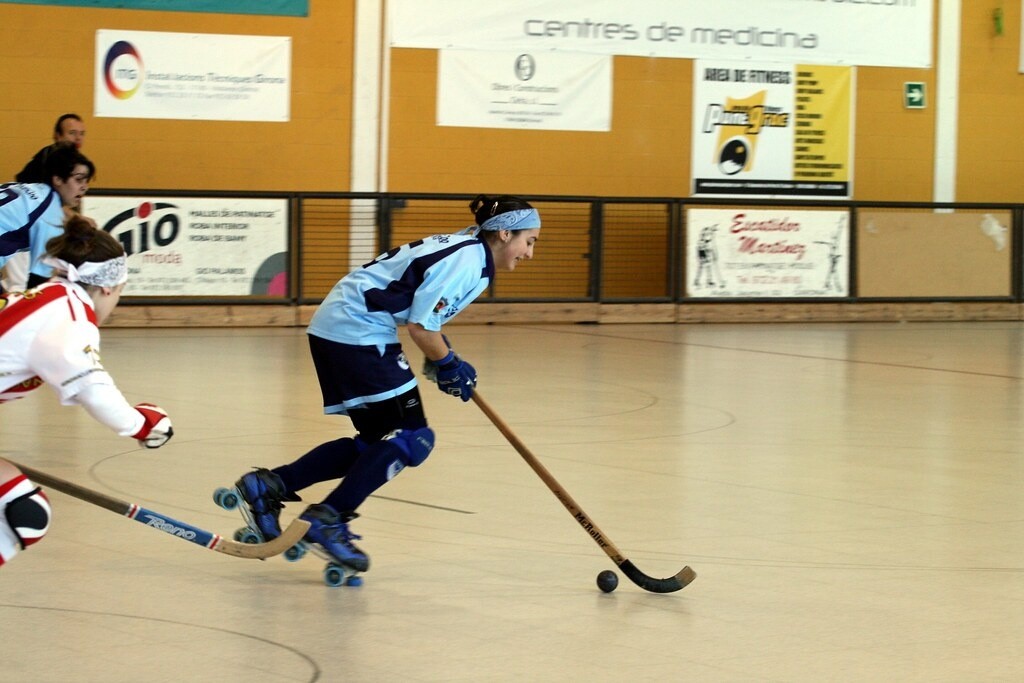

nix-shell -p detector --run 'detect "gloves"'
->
[133,403,174,449]
[422,334,478,401]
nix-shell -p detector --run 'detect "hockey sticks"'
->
[467,385,698,595]
[0,453,314,561]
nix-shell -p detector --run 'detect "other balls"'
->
[596,569,620,594]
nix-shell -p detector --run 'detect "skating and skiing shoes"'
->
[212,466,301,561]
[284,503,370,587]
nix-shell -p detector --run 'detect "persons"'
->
[0,113,175,566]
[212,194,542,588]
